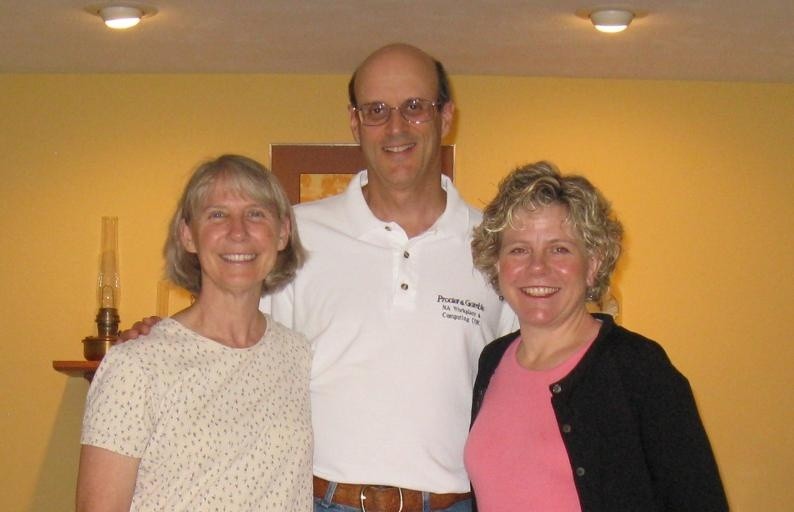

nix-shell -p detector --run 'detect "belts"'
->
[312,475,474,512]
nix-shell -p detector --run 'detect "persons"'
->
[74,151,315,510]
[116,41,520,512]
[462,160,729,511]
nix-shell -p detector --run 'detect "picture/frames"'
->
[267,140,458,212]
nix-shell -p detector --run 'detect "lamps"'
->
[81,3,159,33]
[573,6,647,35]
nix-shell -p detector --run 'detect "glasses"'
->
[351,95,444,129]
